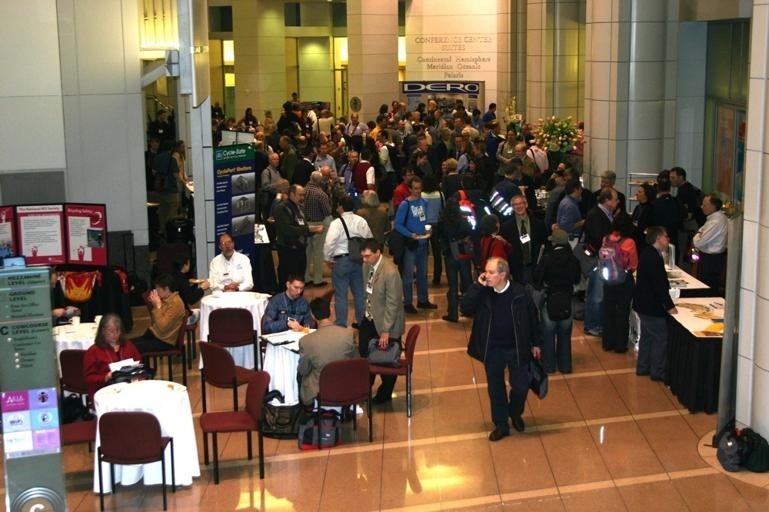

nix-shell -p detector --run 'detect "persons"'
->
[148,91,728,441]
[53,235,253,418]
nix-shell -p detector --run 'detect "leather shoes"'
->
[372,394,391,405]
[404,303,416,313]
[488,425,508,441]
[510,414,524,431]
[418,301,438,309]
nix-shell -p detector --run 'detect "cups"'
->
[94,315,104,324]
[304,324,310,335]
[69,316,79,327]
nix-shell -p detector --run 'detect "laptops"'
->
[2,256,27,267]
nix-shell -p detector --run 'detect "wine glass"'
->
[222,275,235,289]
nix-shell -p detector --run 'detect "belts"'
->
[333,254,349,259]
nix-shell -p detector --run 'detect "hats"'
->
[548,230,568,245]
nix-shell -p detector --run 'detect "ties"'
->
[522,220,529,267]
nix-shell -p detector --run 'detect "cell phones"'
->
[481,276,486,281]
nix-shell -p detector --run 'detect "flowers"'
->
[528,115,586,155]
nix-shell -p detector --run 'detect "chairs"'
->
[365,324,427,421]
[60,420,96,452]
[198,368,270,484]
[96,411,177,512]
[50,265,338,416]
[311,357,377,449]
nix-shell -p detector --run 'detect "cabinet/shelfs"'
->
[628,171,659,215]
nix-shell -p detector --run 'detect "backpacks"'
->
[599,235,630,287]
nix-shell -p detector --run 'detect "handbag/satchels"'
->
[546,284,573,321]
[448,235,476,262]
[388,228,406,266]
[528,359,549,399]
[572,242,600,272]
[347,237,365,262]
[299,412,340,450]
[717,427,769,474]
[260,402,305,440]
[367,340,401,368]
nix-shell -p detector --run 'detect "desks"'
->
[88,379,205,497]
[632,261,727,413]
